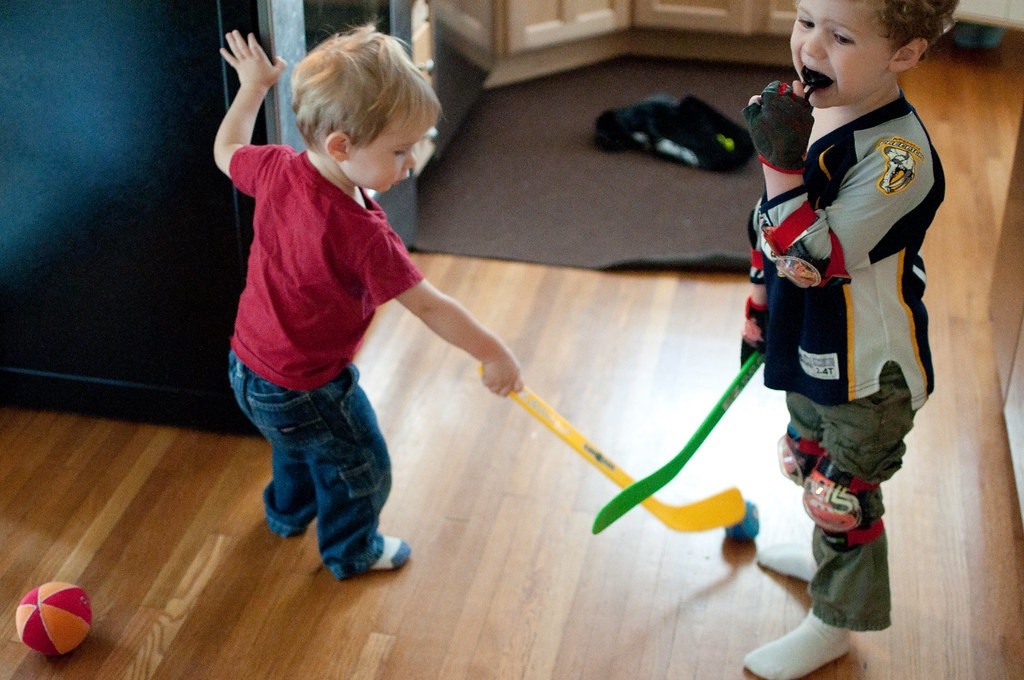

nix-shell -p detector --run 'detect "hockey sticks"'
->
[478,351,767,535]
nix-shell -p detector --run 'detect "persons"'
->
[739,0,960,680]
[212,24,526,580]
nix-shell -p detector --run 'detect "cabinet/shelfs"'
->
[432,0,803,85]
[984,101,1023,517]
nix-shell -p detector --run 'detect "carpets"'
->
[410,56,802,270]
